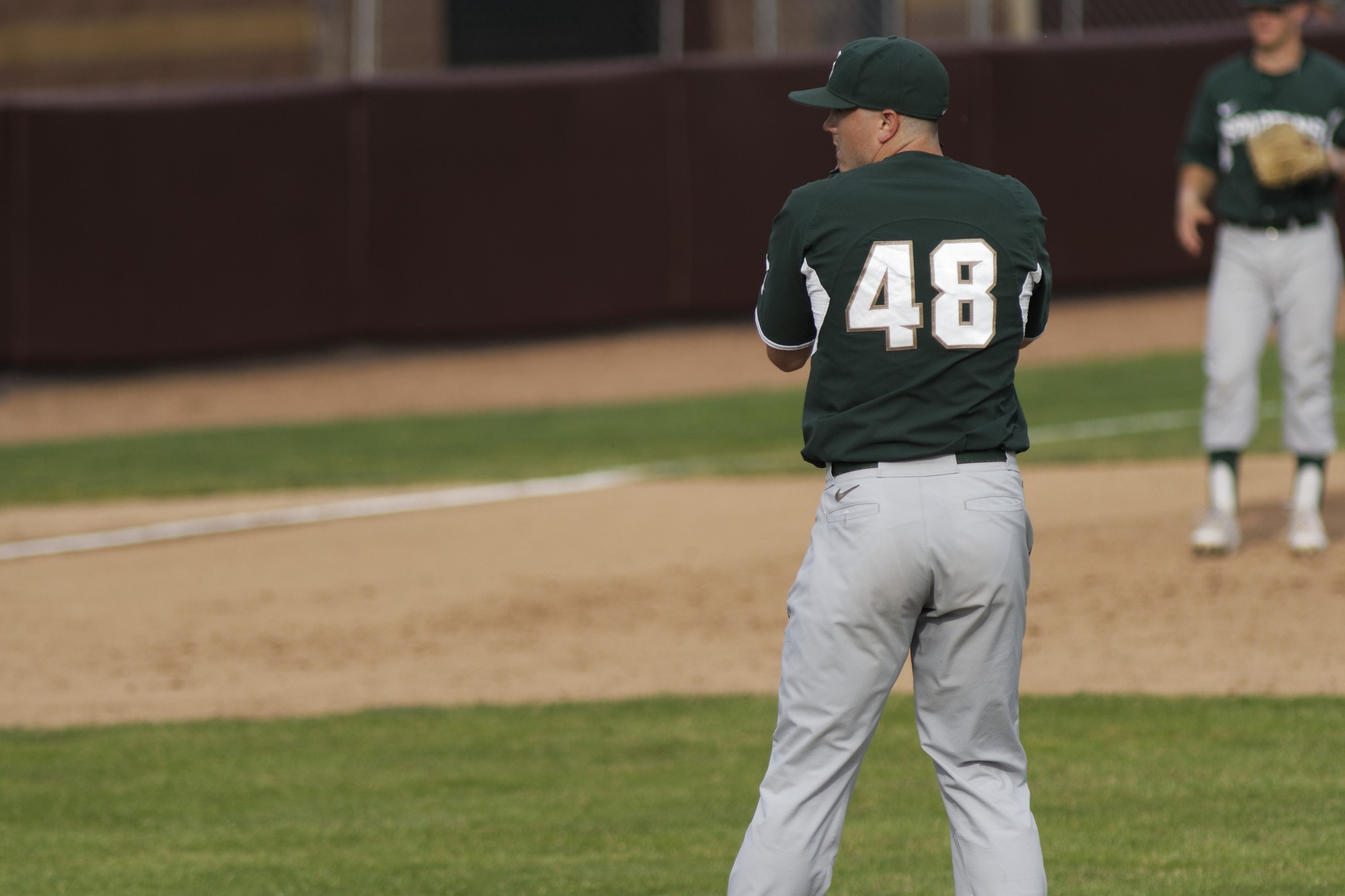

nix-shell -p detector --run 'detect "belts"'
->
[831,449,1006,477]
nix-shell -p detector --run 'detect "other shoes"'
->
[1193,519,1239,550]
[1289,512,1328,550]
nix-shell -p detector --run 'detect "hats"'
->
[788,36,949,121]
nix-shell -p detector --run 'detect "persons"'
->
[1172,1,1345,560]
[724,37,1054,895]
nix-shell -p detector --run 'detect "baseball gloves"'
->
[1247,124,1326,186]
